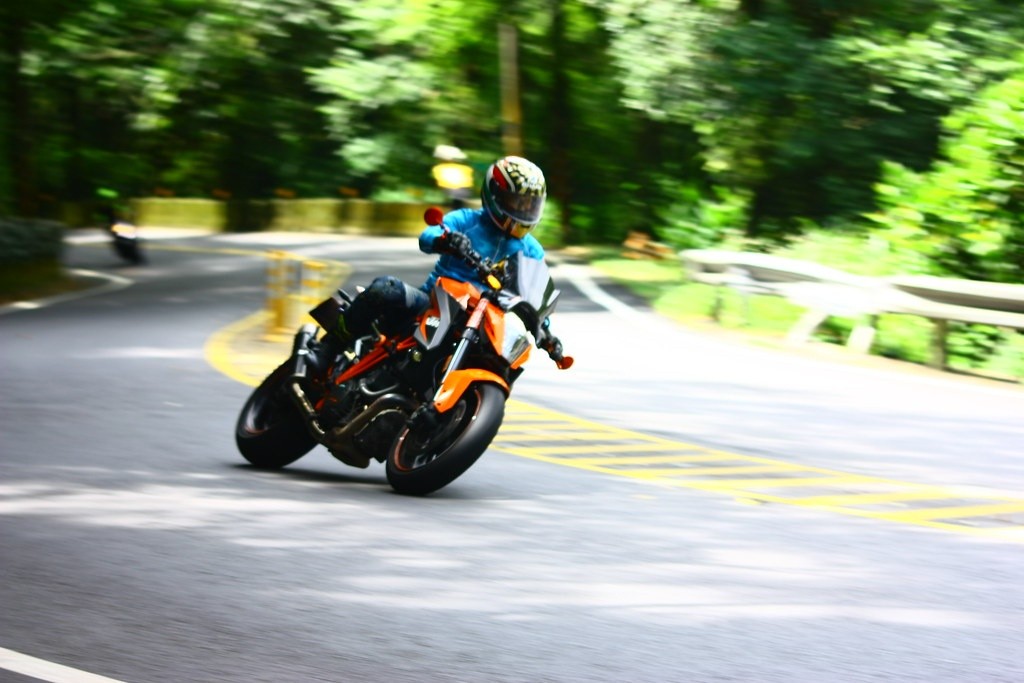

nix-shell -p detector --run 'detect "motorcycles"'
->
[234,207,573,496]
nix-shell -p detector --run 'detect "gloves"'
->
[434,231,470,261]
[542,332,563,362]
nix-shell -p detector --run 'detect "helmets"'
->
[482,154,548,239]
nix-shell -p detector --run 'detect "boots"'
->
[305,316,358,385]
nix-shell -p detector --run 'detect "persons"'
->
[304,156,549,372]
